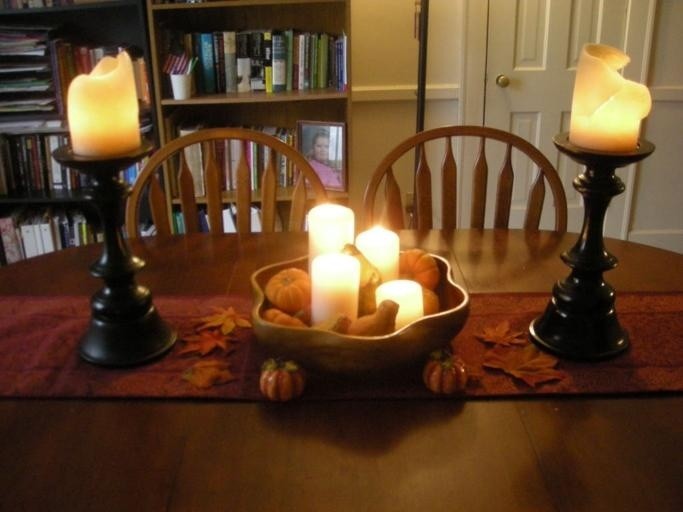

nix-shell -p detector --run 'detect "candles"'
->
[355,224,400,279]
[374,279,425,330]
[567,44,652,151]
[311,252,360,328]
[306,203,356,271]
[67,49,141,156]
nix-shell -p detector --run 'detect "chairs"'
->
[125,128,328,236]
[362,126,568,232]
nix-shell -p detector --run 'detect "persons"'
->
[306,129,339,187]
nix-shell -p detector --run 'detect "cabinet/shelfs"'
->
[0,12,352,265]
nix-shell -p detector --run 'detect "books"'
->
[163,109,299,196]
[0,207,104,265]
[0,0,131,119]
[121,202,263,238]
[152,0,348,95]
[0,131,148,194]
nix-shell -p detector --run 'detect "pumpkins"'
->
[423,356,468,394]
[261,247,442,336]
[258,366,305,402]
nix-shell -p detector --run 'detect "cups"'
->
[168,74,191,100]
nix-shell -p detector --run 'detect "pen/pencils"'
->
[185,56,199,74]
